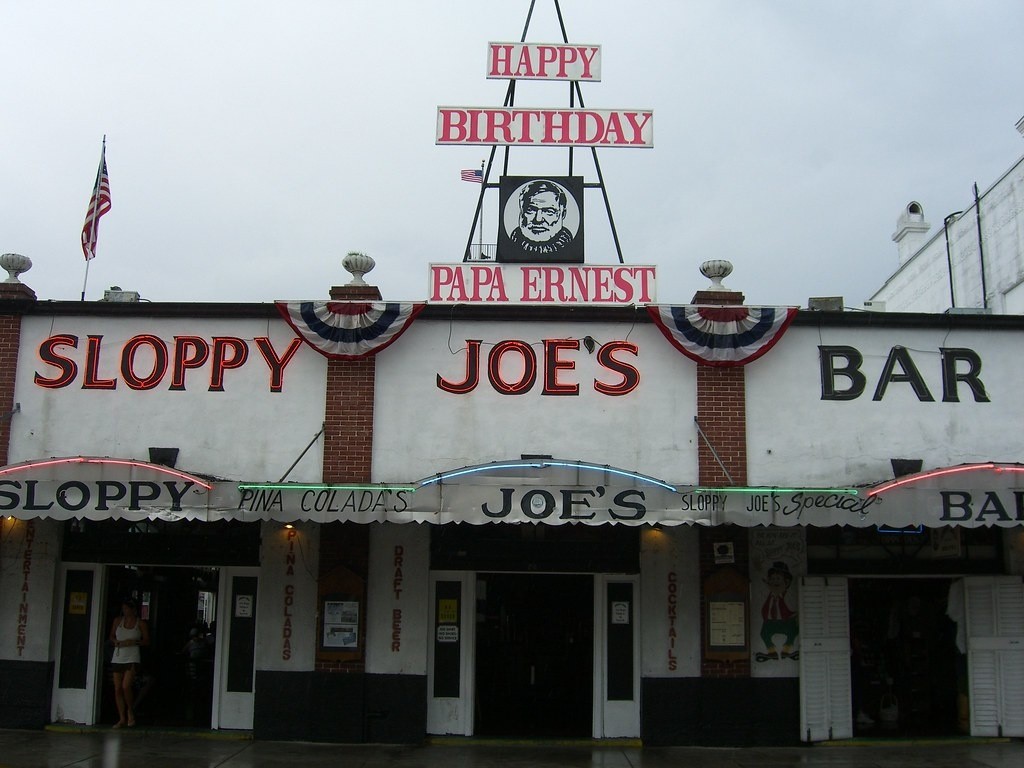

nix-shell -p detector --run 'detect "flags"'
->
[81,140,111,259]
[461,170,483,184]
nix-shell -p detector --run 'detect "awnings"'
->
[0,453,1024,526]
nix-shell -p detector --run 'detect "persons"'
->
[850,623,859,653]
[182,628,205,658]
[110,598,150,730]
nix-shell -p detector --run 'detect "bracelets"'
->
[135,640,140,645]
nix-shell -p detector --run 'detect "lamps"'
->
[654,525,663,531]
[283,522,297,529]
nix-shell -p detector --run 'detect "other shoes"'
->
[112,724,120,728]
[128,717,136,726]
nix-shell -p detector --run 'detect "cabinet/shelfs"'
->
[905,648,931,724]
